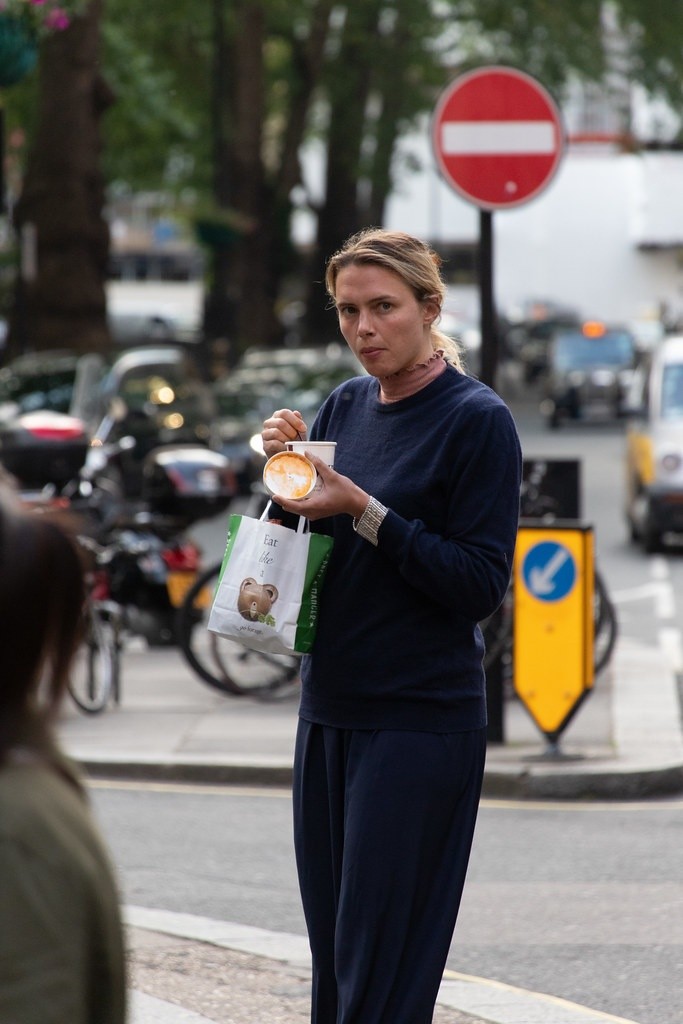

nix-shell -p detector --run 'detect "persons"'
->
[0,467,127,1024]
[262,231,523,1024]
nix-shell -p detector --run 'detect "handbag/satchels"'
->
[206,495,333,657]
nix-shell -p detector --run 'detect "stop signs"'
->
[428,65,566,212]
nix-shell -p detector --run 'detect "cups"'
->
[284,441,337,500]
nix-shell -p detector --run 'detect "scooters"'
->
[42,435,216,648]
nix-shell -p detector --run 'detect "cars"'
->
[621,334,683,553]
[497,297,639,428]
[2,330,376,504]
